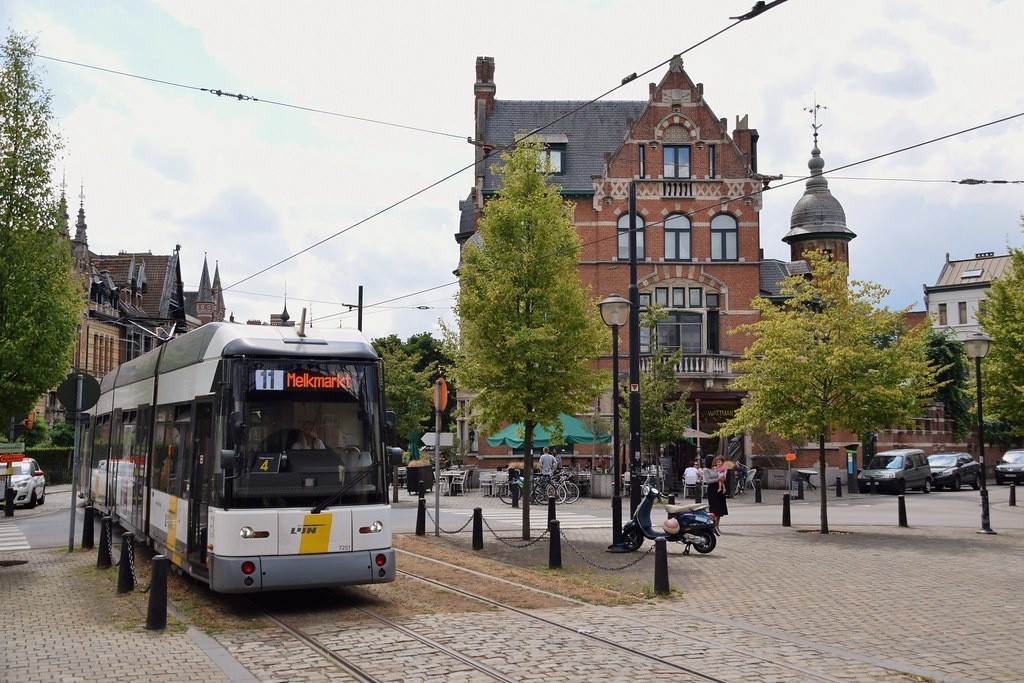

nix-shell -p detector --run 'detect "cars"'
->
[927,452,982,489]
[995,448,1024,485]
[0,458,47,509]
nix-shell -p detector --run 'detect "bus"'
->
[75,308,402,595]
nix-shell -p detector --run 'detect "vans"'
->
[857,448,933,493]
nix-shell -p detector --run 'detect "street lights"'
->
[596,292,634,553]
[964,332,995,534]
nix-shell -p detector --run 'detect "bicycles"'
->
[498,465,581,504]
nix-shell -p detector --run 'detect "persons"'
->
[693,452,747,497]
[683,460,700,498]
[431,451,666,493]
[539,446,558,482]
[715,455,727,494]
[291,418,327,449]
[702,454,728,536]
[550,447,562,477]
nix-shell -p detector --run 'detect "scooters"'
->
[623,474,720,554]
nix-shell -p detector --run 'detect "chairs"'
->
[392,464,665,498]
[681,468,757,499]
[286,429,300,450]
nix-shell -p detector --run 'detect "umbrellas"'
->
[407,418,421,464]
[486,408,613,449]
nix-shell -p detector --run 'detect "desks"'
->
[440,474,460,496]
[797,470,818,491]
[490,474,509,495]
